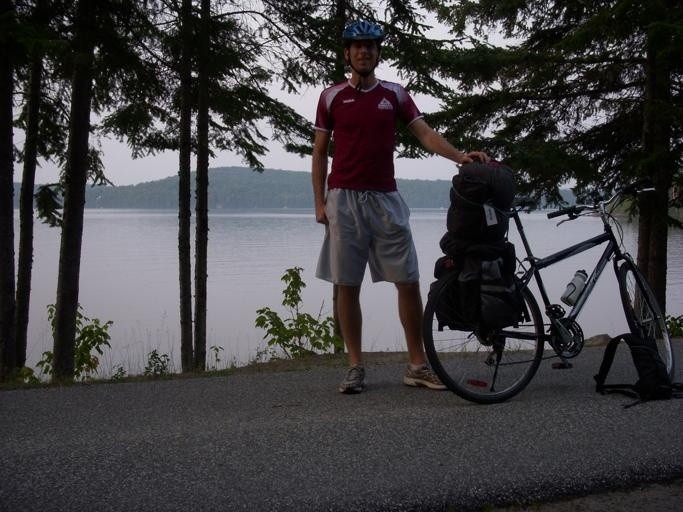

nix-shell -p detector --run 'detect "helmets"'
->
[343,20,385,41]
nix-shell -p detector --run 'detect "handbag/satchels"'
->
[627,334,673,400]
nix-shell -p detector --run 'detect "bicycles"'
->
[421,175,677,405]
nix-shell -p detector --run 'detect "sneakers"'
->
[339,365,365,394]
[403,364,447,390]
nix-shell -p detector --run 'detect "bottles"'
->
[561,267,587,307]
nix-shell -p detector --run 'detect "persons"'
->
[311,21,492,394]
